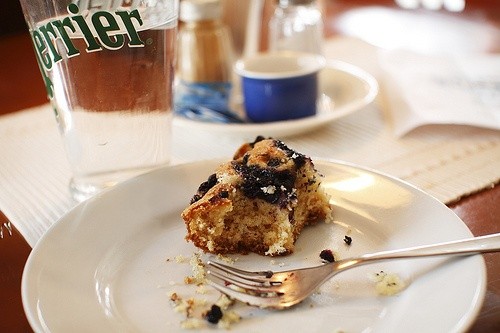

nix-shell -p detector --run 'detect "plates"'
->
[179,63,381,141]
[20,158,486,333]
[339,4,496,55]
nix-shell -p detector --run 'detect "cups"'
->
[20,0,179,192]
[234,54,324,120]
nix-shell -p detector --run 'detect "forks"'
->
[203,234,500,308]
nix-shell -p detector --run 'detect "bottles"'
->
[268,0,325,52]
[176,1,234,91]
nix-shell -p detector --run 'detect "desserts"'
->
[181,135,333,257]
